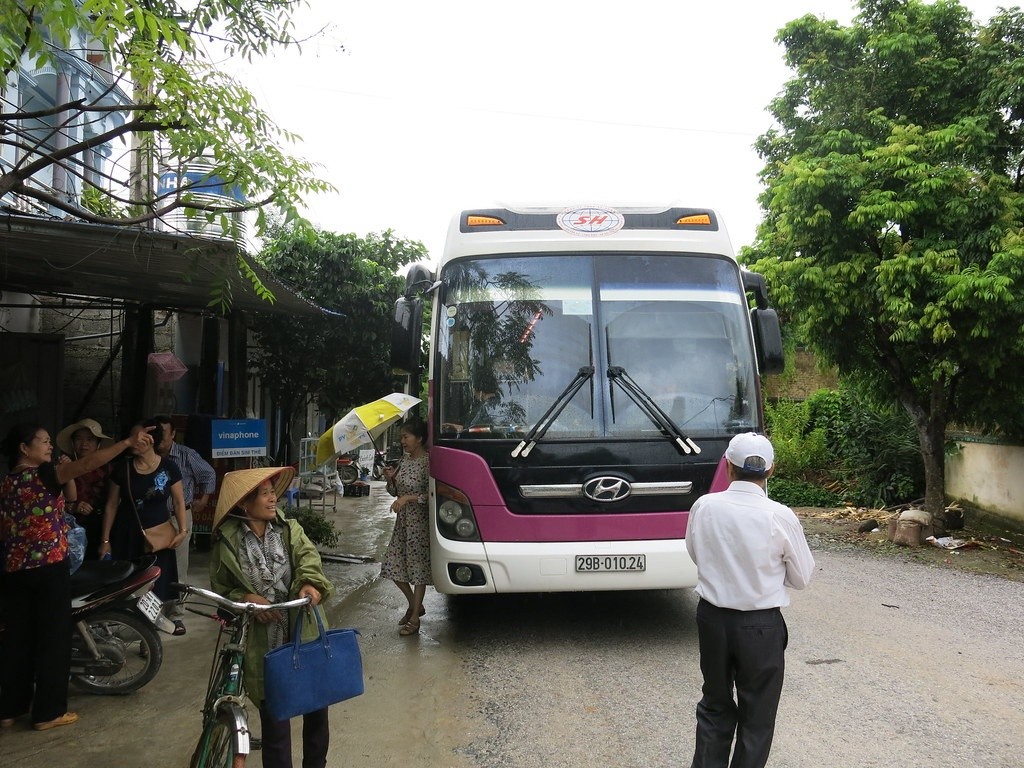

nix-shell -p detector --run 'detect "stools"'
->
[284,488,300,511]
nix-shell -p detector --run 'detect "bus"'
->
[386,205,784,595]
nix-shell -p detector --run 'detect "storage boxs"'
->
[363,485,370,497]
[149,353,188,381]
[343,485,364,497]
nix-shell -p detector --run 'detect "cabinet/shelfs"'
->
[298,438,338,517]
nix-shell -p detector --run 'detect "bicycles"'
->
[159,580,312,768]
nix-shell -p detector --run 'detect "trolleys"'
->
[298,472,337,514]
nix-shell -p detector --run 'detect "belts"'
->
[171,503,192,515]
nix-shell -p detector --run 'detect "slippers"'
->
[33,712,80,730]
[1,713,28,727]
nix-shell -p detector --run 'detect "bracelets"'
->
[100,540,110,543]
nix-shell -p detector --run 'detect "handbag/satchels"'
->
[52,513,88,575]
[142,518,177,553]
[263,602,367,720]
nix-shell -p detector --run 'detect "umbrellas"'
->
[315,392,423,486]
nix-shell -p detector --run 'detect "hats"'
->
[212,466,296,533]
[722,432,774,472]
[56,418,114,455]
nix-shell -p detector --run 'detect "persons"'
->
[56,417,115,561]
[97,418,188,601]
[685,431,814,768]
[152,415,216,635]
[378,416,435,636]
[210,467,335,768]
[1,424,156,730]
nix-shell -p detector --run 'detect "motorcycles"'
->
[372,446,398,480]
[71,551,177,695]
[301,431,363,484]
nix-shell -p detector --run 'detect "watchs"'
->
[179,528,190,533]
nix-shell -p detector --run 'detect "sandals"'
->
[398,605,426,625]
[172,620,186,635]
[399,620,421,635]
